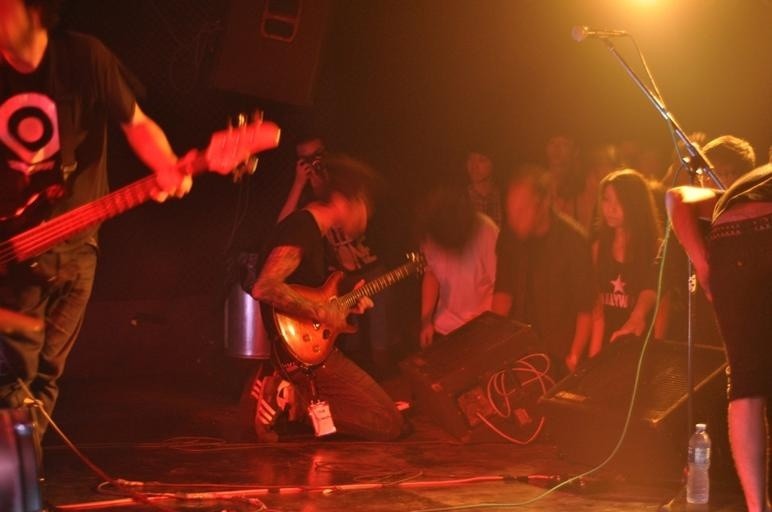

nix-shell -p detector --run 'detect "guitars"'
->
[268,246,430,365]
[1,108,283,279]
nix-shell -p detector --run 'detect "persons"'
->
[665,164,772,511]
[1,1,193,447]
[278,130,330,234]
[546,138,604,220]
[491,163,596,376]
[418,183,501,346]
[587,169,660,359]
[611,134,756,342]
[248,157,404,438]
[460,141,502,227]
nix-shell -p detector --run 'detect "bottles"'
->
[686,423,712,504]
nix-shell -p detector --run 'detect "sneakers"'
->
[238,365,266,424]
[254,377,290,448]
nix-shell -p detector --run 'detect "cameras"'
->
[295,147,328,176]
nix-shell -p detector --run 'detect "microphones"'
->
[570,23,626,46]
[520,465,601,498]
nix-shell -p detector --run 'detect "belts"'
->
[83,235,102,270]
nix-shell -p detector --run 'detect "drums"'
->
[222,258,271,361]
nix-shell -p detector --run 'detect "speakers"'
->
[539,334,737,464]
[390,305,570,449]
